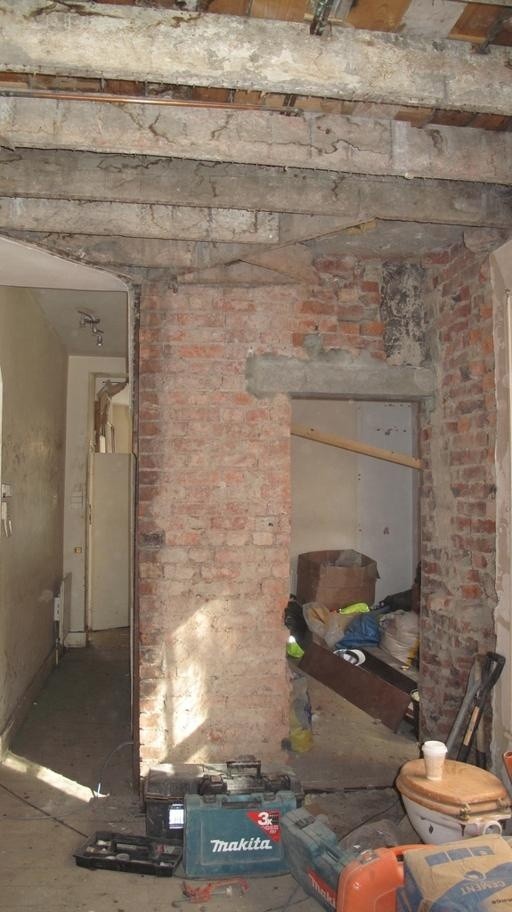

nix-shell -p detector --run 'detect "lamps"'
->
[77,308,105,347]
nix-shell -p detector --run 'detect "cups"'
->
[422,739,449,783]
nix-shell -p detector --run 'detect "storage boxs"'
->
[299,547,382,612]
[394,886,412,912]
[397,832,512,912]
